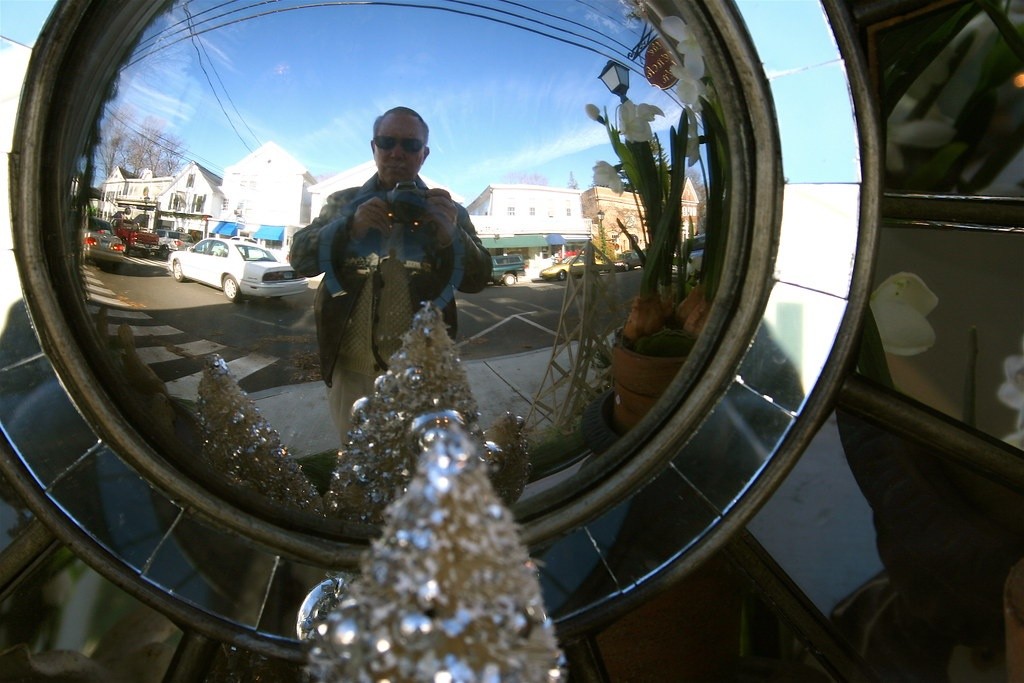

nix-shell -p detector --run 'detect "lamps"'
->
[596,59,632,97]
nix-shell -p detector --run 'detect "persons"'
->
[288,106,495,450]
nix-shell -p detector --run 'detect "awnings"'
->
[548,233,569,246]
[480,235,548,248]
[213,222,237,237]
[252,226,285,242]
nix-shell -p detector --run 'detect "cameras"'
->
[385,180,431,225]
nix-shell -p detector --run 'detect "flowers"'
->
[584,15,732,354]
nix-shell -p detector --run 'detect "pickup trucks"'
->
[110,218,159,259]
[489,255,527,287]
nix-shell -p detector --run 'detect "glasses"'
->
[374,135,427,153]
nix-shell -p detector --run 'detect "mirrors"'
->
[0,0,887,667]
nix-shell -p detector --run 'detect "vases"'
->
[611,326,686,429]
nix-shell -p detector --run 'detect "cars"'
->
[156,230,194,258]
[540,255,605,281]
[607,251,643,272]
[80,217,125,268]
[671,235,705,282]
[167,238,310,307]
